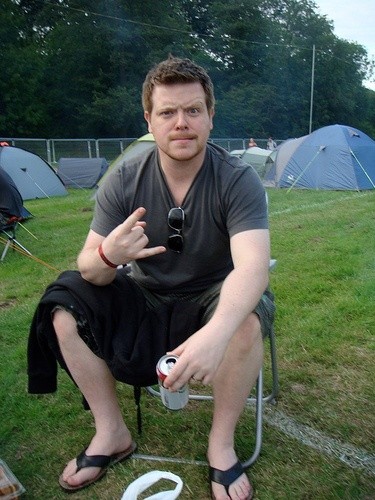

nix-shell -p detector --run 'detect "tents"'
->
[0,142,110,231]
[230,125,375,191]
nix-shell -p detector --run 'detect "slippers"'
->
[58,441,138,491]
[205,446,255,500]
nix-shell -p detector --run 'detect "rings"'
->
[191,376,203,381]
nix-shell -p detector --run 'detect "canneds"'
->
[156,354,190,410]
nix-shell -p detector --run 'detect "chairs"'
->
[132,189,280,469]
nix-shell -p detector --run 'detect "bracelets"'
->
[99,242,119,269]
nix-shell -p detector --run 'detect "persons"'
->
[249,138,256,147]
[49,58,275,500]
[267,137,277,151]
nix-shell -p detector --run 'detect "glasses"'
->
[167,206,184,253]
